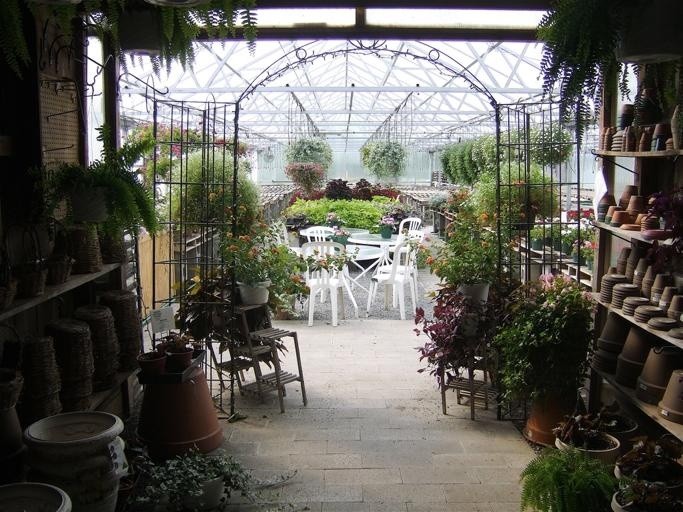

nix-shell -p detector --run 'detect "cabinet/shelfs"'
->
[203,298,307,413]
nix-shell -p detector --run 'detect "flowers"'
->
[572,239,598,258]
[498,272,596,407]
[475,169,559,228]
[219,231,306,298]
[427,207,501,286]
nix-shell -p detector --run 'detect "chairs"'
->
[297,226,352,325]
[367,217,422,319]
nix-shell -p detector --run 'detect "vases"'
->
[1,122,226,509]
[520,381,588,448]
[236,279,271,307]
[571,255,595,270]
[455,278,490,308]
[510,206,538,231]
[593,105,682,425]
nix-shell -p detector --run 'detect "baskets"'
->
[2,219,129,309]
[22,291,142,455]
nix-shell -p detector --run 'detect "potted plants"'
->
[533,2,681,150]
[528,225,585,254]
[517,410,681,512]
[0,1,256,81]
